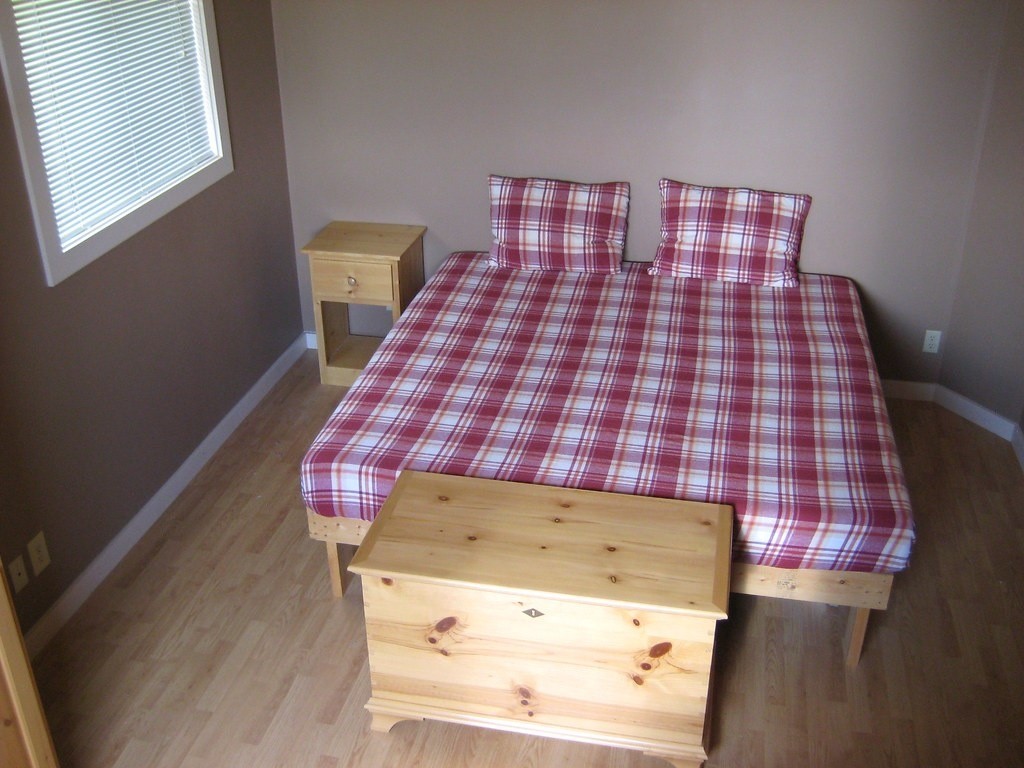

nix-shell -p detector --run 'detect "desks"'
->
[348,469,735,768]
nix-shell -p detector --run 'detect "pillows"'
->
[647,178,811,288]
[484,174,631,275]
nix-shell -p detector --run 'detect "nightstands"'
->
[301,221,427,386]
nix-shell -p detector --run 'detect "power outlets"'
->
[27,531,51,577]
[923,330,941,353]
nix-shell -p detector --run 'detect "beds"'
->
[302,251,917,667]
